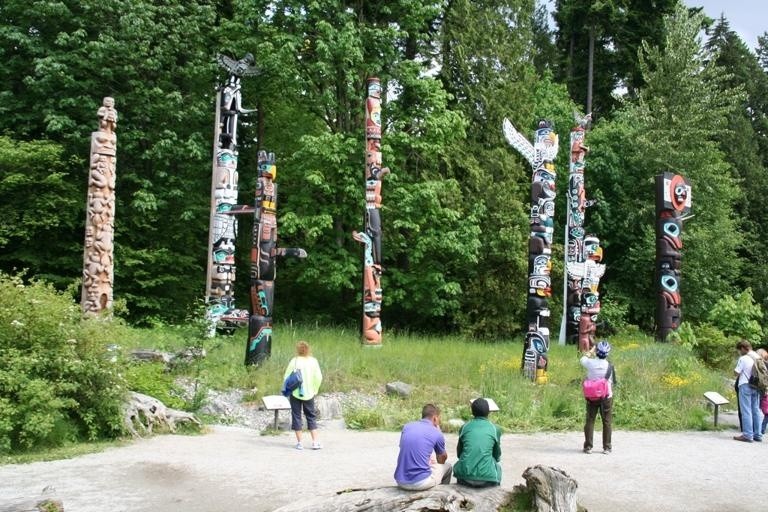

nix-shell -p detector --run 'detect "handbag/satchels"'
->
[582,378,611,402]
[281,368,304,396]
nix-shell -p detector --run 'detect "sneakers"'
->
[582,445,613,456]
[311,442,322,450]
[293,442,304,450]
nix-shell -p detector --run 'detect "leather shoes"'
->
[732,433,766,443]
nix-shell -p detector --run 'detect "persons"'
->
[284,341,323,450]
[454,398,502,489]
[580,341,617,454]
[395,404,453,491]
[734,341,768,442]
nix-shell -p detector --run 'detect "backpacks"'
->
[747,354,768,397]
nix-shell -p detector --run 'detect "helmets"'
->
[594,339,612,360]
